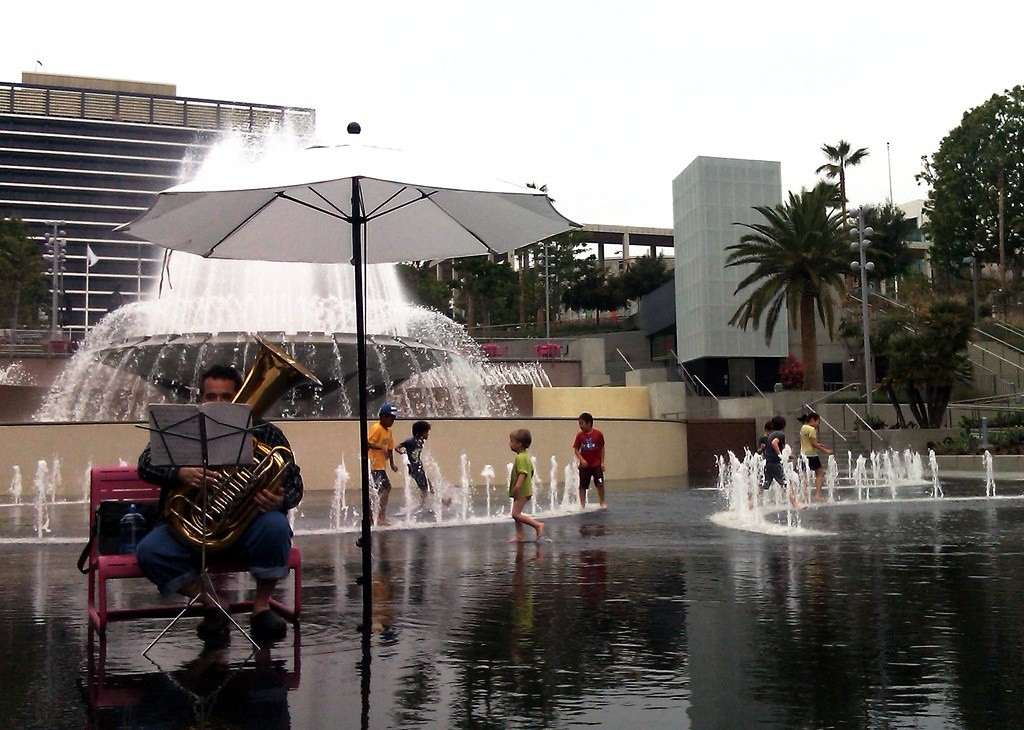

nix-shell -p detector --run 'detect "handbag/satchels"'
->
[77,502,153,573]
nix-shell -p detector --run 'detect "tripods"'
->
[134,401,270,653]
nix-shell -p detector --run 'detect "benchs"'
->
[89,468,301,633]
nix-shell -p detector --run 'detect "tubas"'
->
[164,335,323,548]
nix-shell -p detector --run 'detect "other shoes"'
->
[197,608,231,632]
[250,610,288,633]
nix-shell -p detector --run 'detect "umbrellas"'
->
[112,120,585,642]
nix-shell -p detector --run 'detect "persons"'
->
[796,412,833,501]
[757,420,773,459]
[507,429,545,544]
[748,414,809,511]
[396,420,452,511]
[365,405,400,527]
[131,363,305,641]
[573,411,608,510]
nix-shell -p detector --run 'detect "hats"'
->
[380,402,401,417]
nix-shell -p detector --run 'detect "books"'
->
[149,403,253,466]
